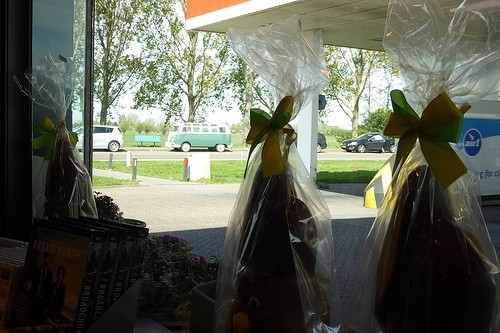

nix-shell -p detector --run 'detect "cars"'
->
[340,132,397,153]
[289,130,327,152]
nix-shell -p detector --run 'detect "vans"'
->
[68,124,124,152]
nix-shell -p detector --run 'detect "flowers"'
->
[144,234,216,295]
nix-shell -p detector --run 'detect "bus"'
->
[168,122,231,152]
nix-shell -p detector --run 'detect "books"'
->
[0,214,150,333]
